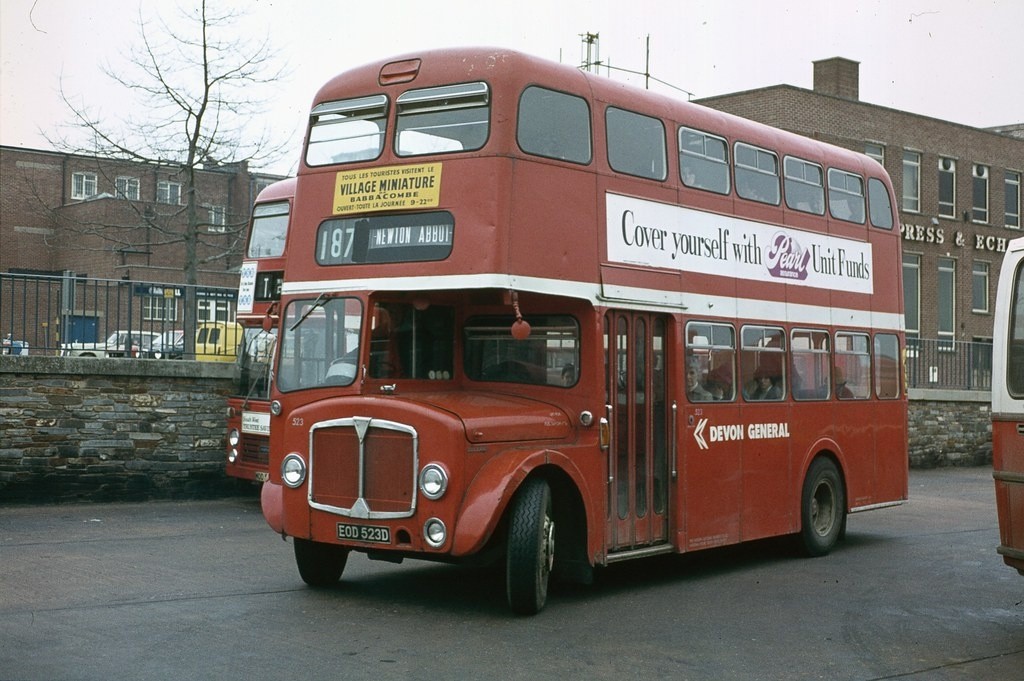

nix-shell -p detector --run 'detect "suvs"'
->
[59,327,162,358]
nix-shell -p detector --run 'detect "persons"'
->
[825,367,853,399]
[682,164,705,189]
[749,364,783,400]
[561,362,577,387]
[687,357,713,401]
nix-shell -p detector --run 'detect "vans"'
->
[195,321,243,361]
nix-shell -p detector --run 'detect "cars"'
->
[129,330,189,360]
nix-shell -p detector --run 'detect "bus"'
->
[259,42,912,617]
[989,238,1024,578]
[225,176,381,487]
